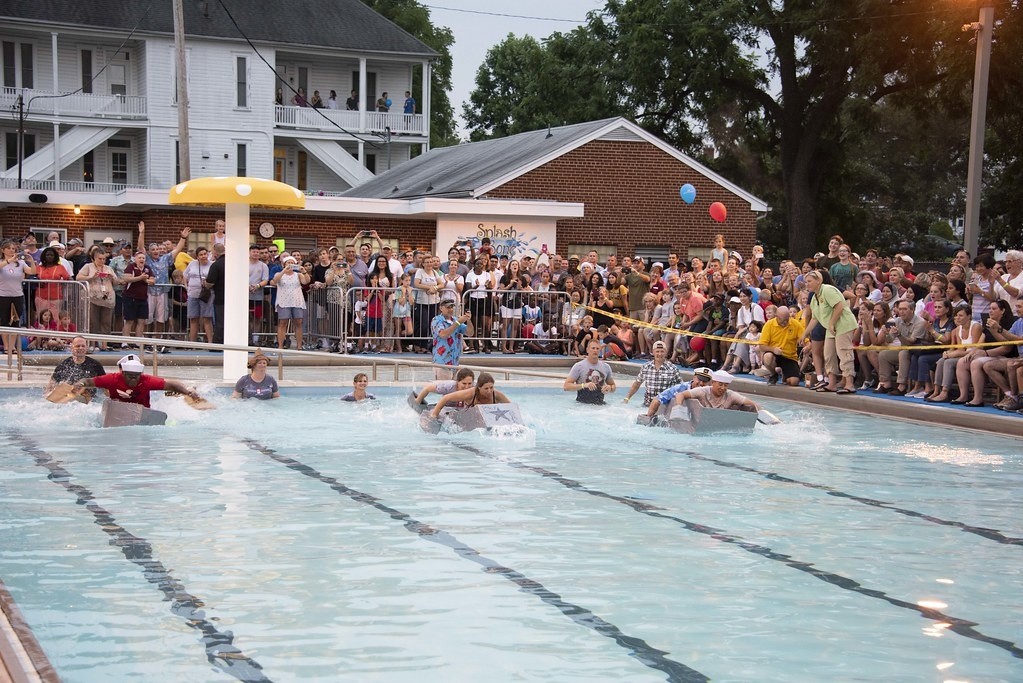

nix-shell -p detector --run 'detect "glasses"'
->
[441,299,454,305]
[73,238,81,244]
[269,250,278,253]
[344,244,1023,324]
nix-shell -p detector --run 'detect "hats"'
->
[383,243,392,249]
[856,270,878,286]
[631,256,644,263]
[117,355,140,366]
[730,252,743,264]
[897,256,914,266]
[653,340,667,351]
[122,361,145,373]
[694,367,714,382]
[521,254,531,260]
[247,349,271,369]
[728,296,742,304]
[328,245,340,252]
[568,258,580,262]
[712,370,734,384]
[25,232,135,255]
[282,256,297,264]
[439,298,455,307]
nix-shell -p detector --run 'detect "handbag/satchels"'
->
[199,285,211,303]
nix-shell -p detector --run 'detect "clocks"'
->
[258,222,276,238]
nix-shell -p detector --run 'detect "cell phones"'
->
[337,264,346,268]
[290,264,300,270]
[969,283,976,294]
[592,289,599,295]
[363,231,371,236]
[98,273,108,278]
[458,241,468,246]
[755,254,764,258]
[622,268,631,275]
[981,313,991,328]
[17,254,26,260]
[542,244,547,253]
[885,322,895,329]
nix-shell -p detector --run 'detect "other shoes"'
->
[4,330,1023,417]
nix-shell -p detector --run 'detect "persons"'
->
[0,220,1023,420]
[233,348,279,400]
[431,295,474,380]
[346,90,359,111]
[291,87,310,107]
[312,90,323,108]
[404,91,416,129]
[326,90,339,110]
[342,373,376,402]
[376,92,390,112]
[276,87,285,110]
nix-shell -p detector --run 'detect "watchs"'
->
[997,327,1003,334]
[981,292,986,297]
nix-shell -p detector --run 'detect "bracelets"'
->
[731,316,736,320]
[181,236,186,239]
[346,271,352,276]
[997,266,1003,271]
[302,270,307,274]
[604,296,607,301]
[624,398,630,401]
[897,333,902,338]
[635,272,640,277]
[6,259,10,264]
[864,329,869,333]
[508,285,510,288]
[581,382,585,390]
[377,236,380,241]
[935,334,943,341]
[454,280,459,284]
[455,320,461,327]
[257,283,262,288]
[433,285,439,290]
[1002,282,1010,289]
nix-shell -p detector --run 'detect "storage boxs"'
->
[450,403,525,436]
[101,398,168,427]
[665,398,759,436]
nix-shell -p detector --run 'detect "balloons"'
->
[681,183,695,204]
[710,202,727,223]
[386,99,392,107]
